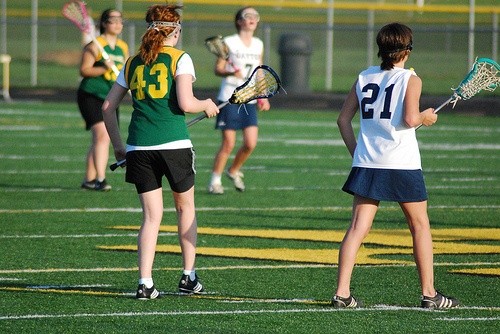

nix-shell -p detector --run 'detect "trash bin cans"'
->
[278,34,312,94]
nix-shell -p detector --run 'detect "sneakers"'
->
[135,283,162,301]
[332,293,364,309]
[178,273,205,295]
[420,290,459,309]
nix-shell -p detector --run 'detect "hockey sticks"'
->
[204,34,264,106]
[109,64,282,171]
[416,57,500,131]
[60,0,132,98]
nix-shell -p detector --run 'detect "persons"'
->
[77,9,130,191]
[330,23,459,310]
[102,4,219,301]
[208,6,271,194]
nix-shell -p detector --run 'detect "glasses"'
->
[103,16,125,25]
[239,10,260,22]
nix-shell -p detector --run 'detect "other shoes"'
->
[226,168,246,192]
[81,179,112,191]
[207,183,224,195]
